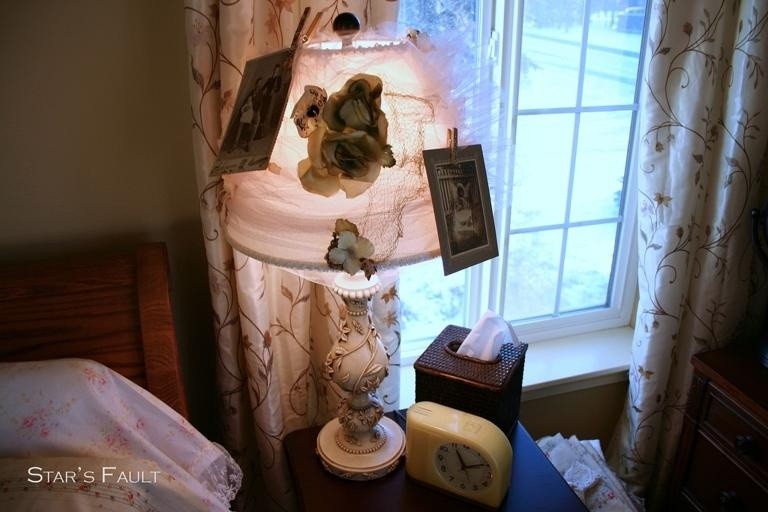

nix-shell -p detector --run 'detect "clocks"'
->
[404,400,513,507]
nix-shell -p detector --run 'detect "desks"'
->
[281,405,587,511]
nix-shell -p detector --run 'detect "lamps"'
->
[217,11,473,484]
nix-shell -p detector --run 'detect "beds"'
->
[0,237,221,512]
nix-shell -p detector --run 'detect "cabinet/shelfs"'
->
[660,340,767,512]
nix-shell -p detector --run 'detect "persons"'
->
[216,76,263,154]
[239,96,256,144]
[453,185,474,227]
[251,62,281,143]
